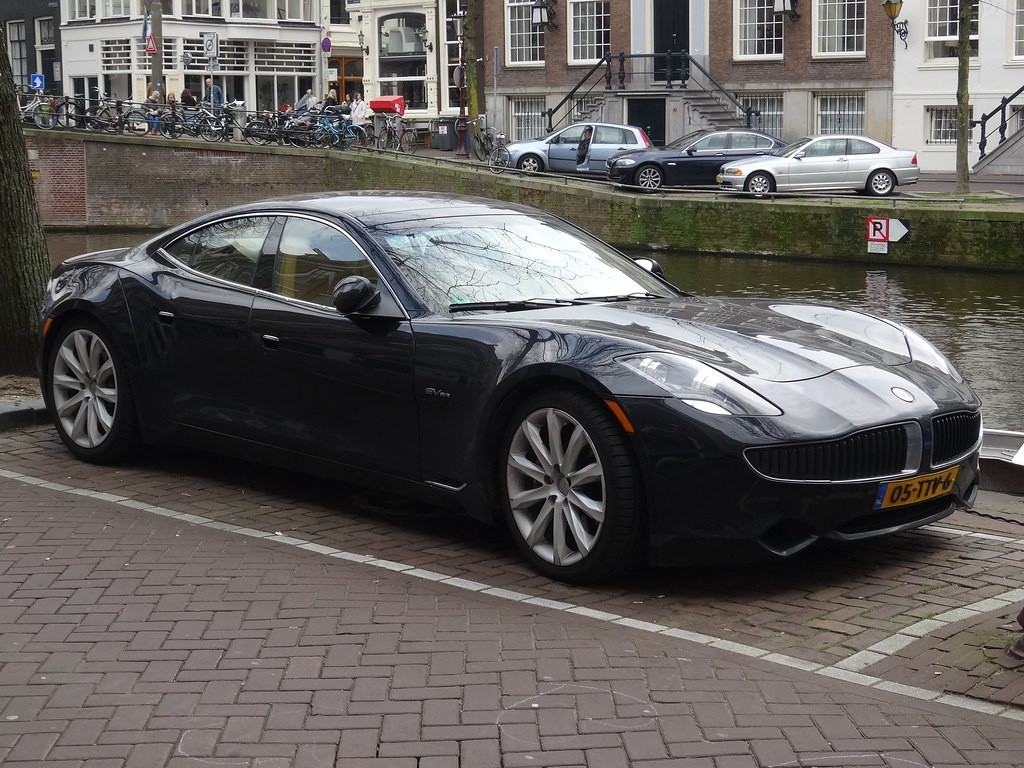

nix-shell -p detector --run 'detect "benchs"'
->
[124,107,206,133]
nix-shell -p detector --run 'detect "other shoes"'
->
[151,128,157,135]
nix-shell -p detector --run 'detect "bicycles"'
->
[465,115,494,162]
[376,111,418,154]
[484,126,510,175]
[14,82,377,152]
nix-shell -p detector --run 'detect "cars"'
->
[499,121,654,182]
[604,125,792,194]
[714,132,921,199]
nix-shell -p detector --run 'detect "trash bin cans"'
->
[232,101,247,141]
[435,114,456,150]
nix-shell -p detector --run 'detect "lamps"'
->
[882,0,909,49]
[416,23,432,52]
[530,0,559,31]
[772,0,801,22]
[358,30,369,54]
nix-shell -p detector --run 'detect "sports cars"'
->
[34,186,985,589]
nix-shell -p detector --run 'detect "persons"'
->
[349,92,366,128]
[141,91,162,135]
[204,78,223,125]
[163,92,177,135]
[341,94,352,106]
[306,93,317,110]
[180,89,197,110]
[326,89,338,105]
[577,129,591,164]
